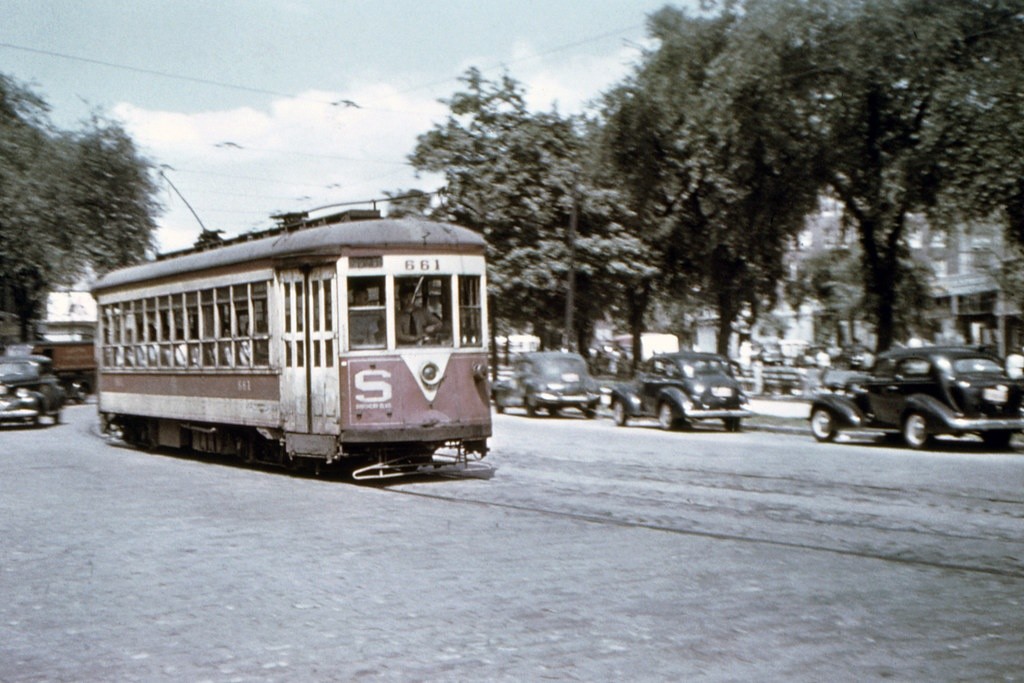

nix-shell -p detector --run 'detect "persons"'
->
[104,321,268,365]
[395,284,442,344]
[353,289,369,305]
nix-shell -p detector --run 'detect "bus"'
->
[90,209,494,476]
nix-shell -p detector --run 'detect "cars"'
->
[489,352,601,420]
[0,355,68,428]
[609,352,757,432]
[805,346,1024,449]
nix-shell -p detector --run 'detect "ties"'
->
[410,312,417,336]
